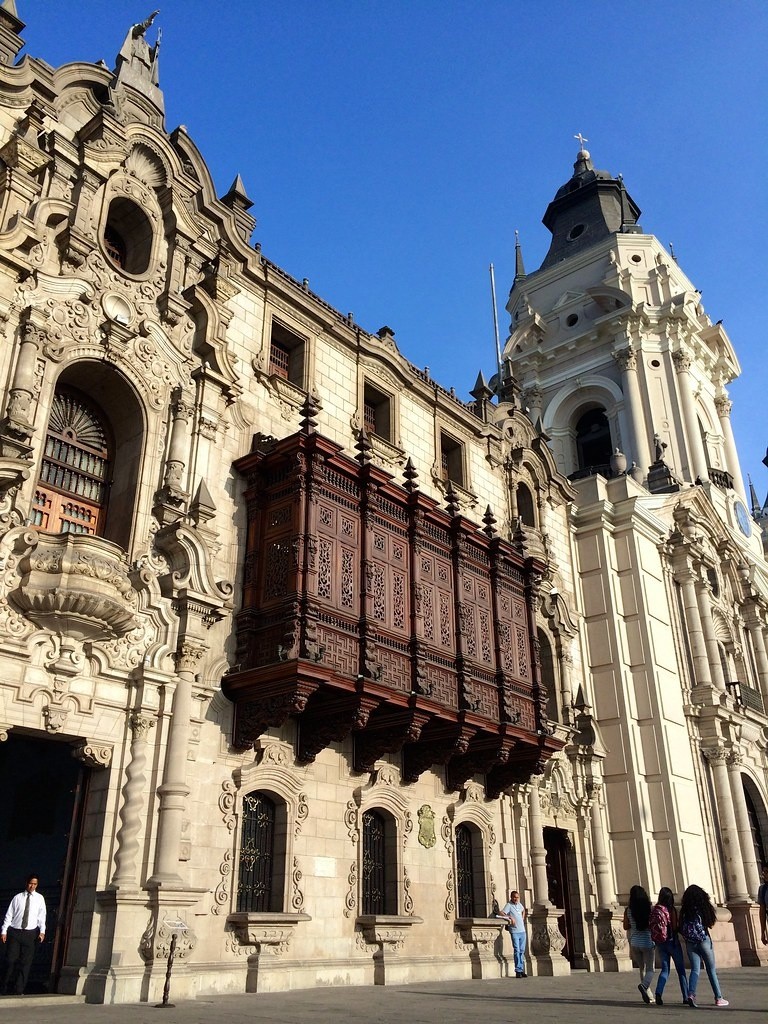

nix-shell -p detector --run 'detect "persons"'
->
[623,885,729,1008]
[654,433,665,460]
[758,862,768,946]
[501,891,528,978]
[1,874,46,995]
[122,19,161,85]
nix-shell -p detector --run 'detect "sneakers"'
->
[688,996,697,1007]
[715,998,729,1006]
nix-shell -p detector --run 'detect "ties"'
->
[22,892,31,929]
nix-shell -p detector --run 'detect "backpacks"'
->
[680,909,706,942]
[649,905,673,943]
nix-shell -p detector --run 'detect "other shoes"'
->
[682,999,688,1004]
[521,972,527,977]
[638,984,653,1004]
[656,993,663,1005]
[516,972,522,978]
